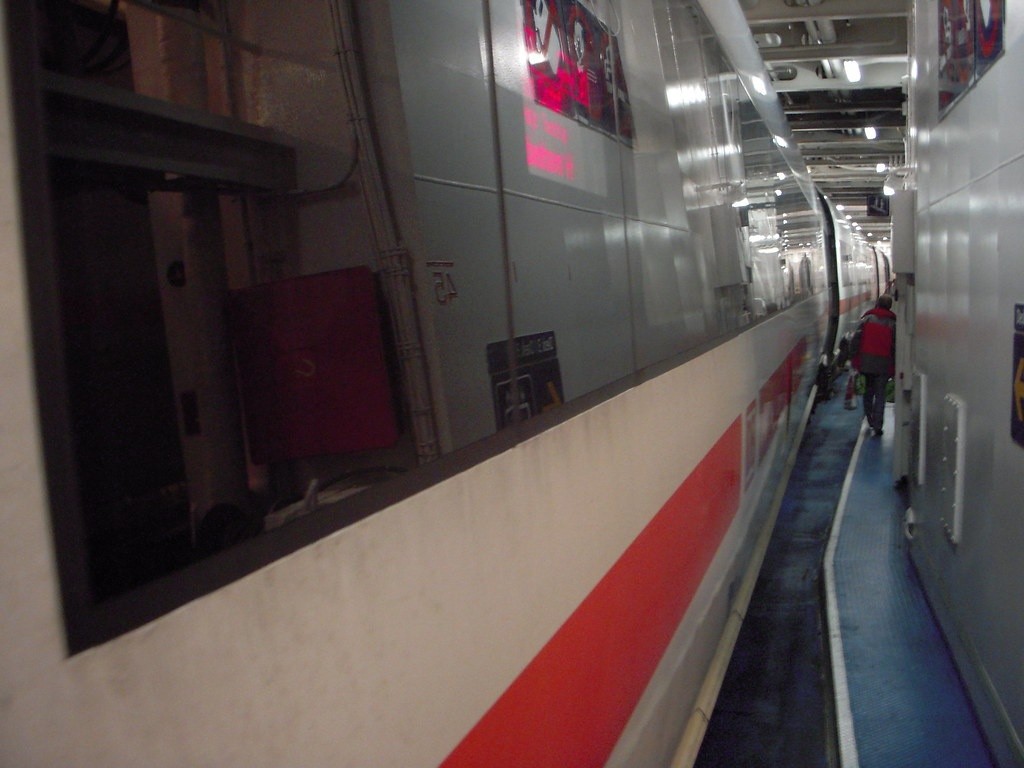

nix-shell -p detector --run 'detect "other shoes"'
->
[875,430,883,435]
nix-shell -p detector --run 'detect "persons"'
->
[851,294,897,437]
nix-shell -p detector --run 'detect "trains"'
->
[0,0,895,768]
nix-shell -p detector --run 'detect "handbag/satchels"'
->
[855,373,866,395]
[886,380,895,403]
